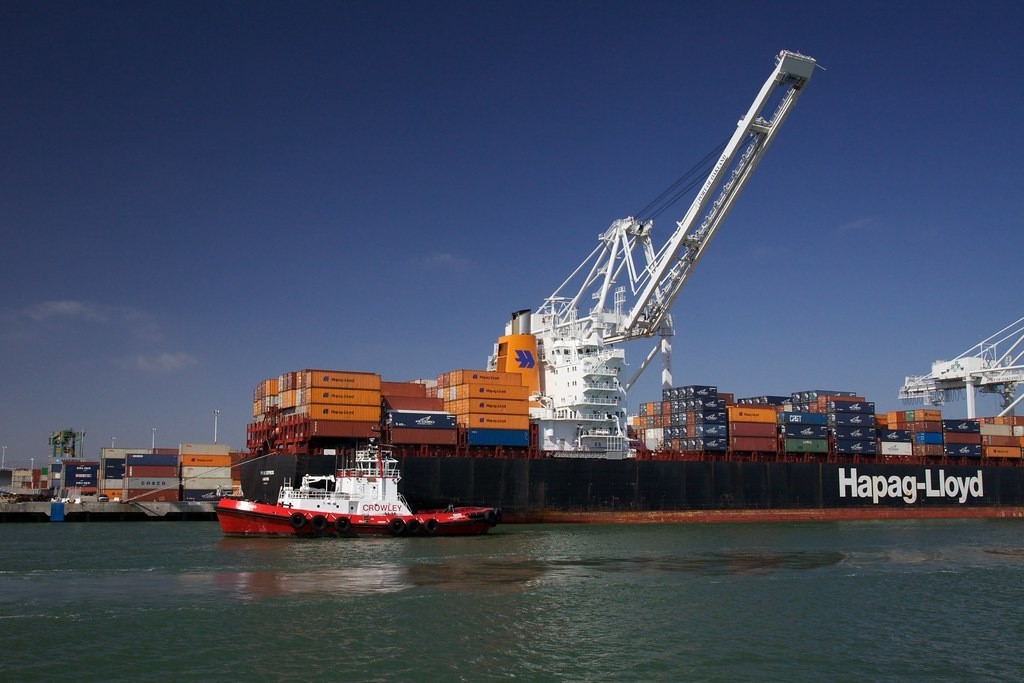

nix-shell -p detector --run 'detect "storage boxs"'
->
[628,384,1024,459]
[11,369,530,502]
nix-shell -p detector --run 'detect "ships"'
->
[234,305,1024,526]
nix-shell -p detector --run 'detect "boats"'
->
[211,435,505,536]
